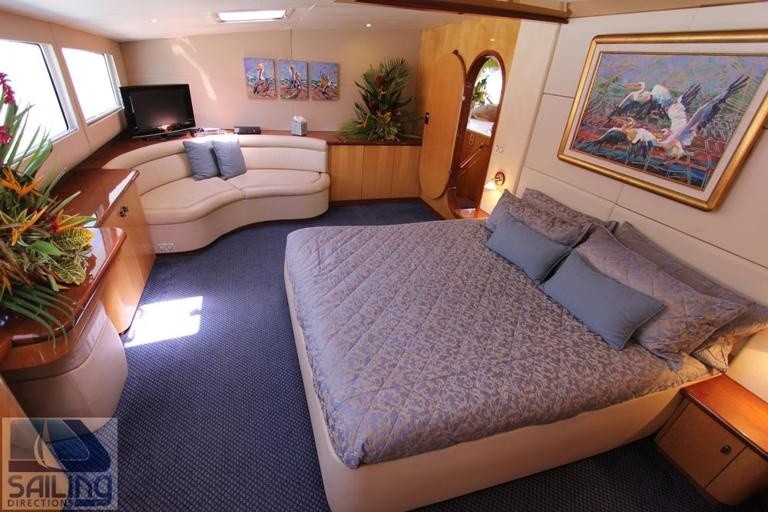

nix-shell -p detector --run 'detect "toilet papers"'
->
[291,116,307,136]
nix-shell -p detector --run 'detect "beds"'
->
[282,217,753,511]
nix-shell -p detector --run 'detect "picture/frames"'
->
[556,28,768,213]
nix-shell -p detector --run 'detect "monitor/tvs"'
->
[119,83,197,142]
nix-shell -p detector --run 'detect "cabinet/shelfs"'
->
[99,182,157,336]
[328,145,422,206]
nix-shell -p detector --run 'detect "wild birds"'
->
[586,72,751,165]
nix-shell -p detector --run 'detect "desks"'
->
[6,226,130,447]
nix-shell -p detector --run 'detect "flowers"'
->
[334,56,422,144]
[0,72,97,351]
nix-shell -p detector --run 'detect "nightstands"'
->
[652,374,768,507]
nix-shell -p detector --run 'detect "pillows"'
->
[521,188,619,248]
[613,221,768,374]
[211,134,247,181]
[553,223,749,375]
[183,135,221,181]
[481,189,593,255]
[484,210,572,285]
[536,248,664,351]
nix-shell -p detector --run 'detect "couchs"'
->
[101,133,330,255]
